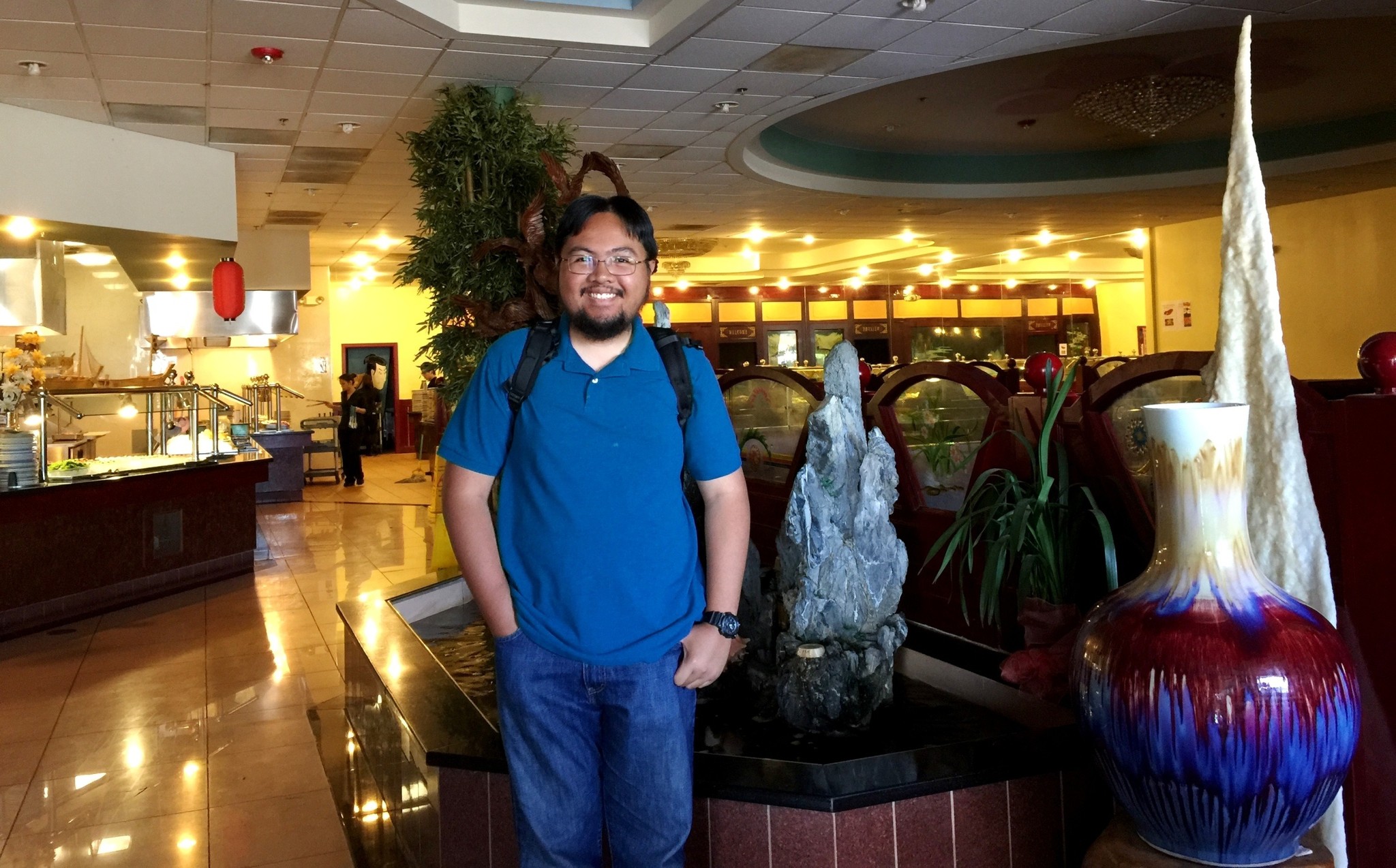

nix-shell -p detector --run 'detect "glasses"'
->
[554,251,648,277]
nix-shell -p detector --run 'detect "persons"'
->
[420,362,438,389]
[324,373,369,487]
[433,196,754,868]
[425,377,453,475]
[152,414,189,455]
[357,375,382,456]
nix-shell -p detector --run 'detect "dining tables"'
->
[754,344,1144,393]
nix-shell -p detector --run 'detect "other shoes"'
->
[355,477,363,484]
[343,480,354,486]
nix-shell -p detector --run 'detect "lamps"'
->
[316,355,329,374]
[299,294,325,305]
[17,398,42,427]
[167,392,190,410]
[116,393,139,420]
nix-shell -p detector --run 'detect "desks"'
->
[2,426,314,643]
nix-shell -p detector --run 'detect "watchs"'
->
[702,611,740,640]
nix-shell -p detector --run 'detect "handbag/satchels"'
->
[347,405,357,428]
[367,409,379,420]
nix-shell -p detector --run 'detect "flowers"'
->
[0,330,48,414]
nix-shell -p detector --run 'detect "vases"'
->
[4,411,23,434]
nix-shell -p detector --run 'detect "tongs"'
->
[306,399,326,408]
[348,406,358,429]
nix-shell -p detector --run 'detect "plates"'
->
[274,410,291,421]
